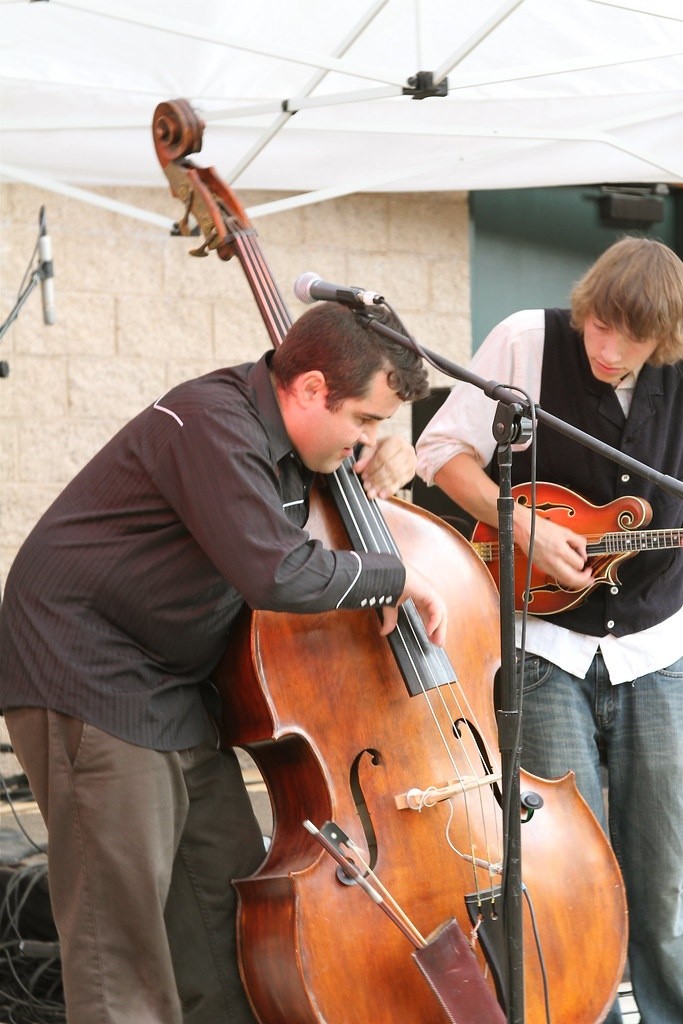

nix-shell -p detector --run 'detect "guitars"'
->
[471,479,682,619]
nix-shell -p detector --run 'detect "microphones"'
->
[40,210,55,325]
[293,271,386,306]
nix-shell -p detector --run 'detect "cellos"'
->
[154,101,629,1024]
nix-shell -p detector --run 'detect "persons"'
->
[1,299,448,1023]
[415,236,683,1024]
